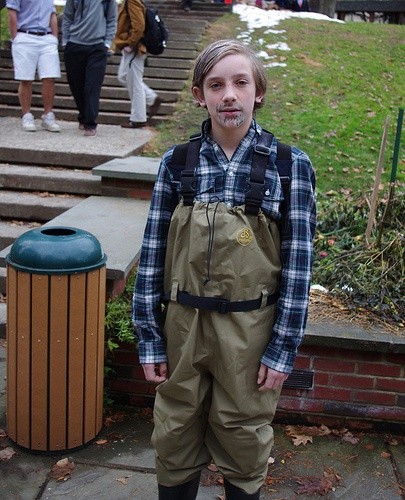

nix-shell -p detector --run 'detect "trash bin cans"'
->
[4,225,105,454]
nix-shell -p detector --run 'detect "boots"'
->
[223,476,260,500]
[157,474,202,500]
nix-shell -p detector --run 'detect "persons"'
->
[61,0,118,136]
[182,0,193,11]
[6,0,61,132]
[112,0,161,128]
[132,41,317,500]
[213,0,310,12]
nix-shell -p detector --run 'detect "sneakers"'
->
[41,112,60,131]
[22,113,36,131]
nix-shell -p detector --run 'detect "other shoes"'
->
[148,96,163,119]
[121,118,147,128]
[78,121,84,130]
[83,128,97,138]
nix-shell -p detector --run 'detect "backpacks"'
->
[123,0,168,54]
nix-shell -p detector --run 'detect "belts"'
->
[17,29,52,36]
